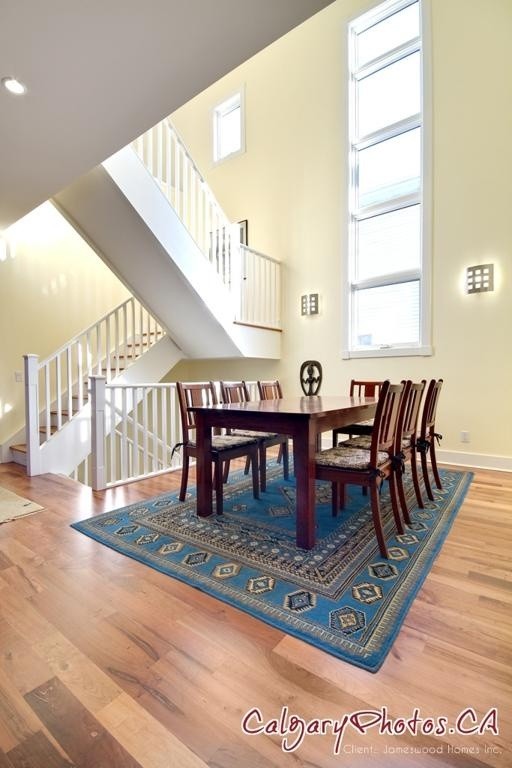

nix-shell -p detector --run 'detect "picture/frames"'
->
[208,219,249,281]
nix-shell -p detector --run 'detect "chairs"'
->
[314,380,441,558]
[175,381,294,515]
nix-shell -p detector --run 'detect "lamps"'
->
[465,263,495,294]
[301,293,319,316]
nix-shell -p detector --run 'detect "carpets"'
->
[0,486,48,525]
[69,449,475,674]
